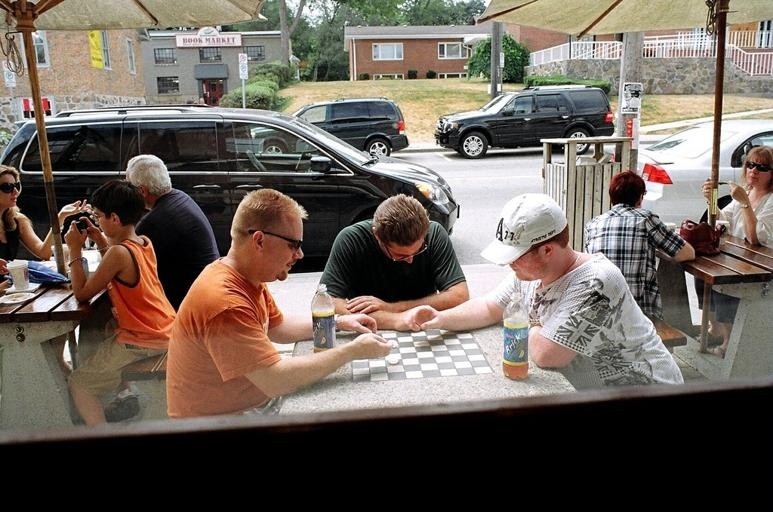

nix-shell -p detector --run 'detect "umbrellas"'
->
[478,2,773,357]
[2,259,71,285]
[0,1,267,370]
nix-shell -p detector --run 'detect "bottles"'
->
[310,284,336,352]
[501,293,529,380]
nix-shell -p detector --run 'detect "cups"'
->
[5,260,29,291]
[50,244,69,271]
[663,221,675,232]
[716,220,730,247]
[80,257,88,280]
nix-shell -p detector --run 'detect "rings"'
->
[80,210,84,213]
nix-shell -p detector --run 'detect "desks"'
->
[652,228,773,383]
[280,321,573,410]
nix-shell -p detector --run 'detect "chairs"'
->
[239,133,267,171]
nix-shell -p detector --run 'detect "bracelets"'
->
[58,224,63,234]
[96,243,110,252]
[68,257,85,267]
[739,202,753,209]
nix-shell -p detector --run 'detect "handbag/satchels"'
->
[679,217,726,257]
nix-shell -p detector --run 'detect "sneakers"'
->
[101,392,139,422]
[693,330,728,358]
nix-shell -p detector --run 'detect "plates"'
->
[0,293,34,305]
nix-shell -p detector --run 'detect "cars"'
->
[634,119,773,224]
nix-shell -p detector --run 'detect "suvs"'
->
[0,105,460,275]
[434,84,616,157]
[251,97,410,158]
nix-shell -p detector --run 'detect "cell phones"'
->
[74,218,88,233]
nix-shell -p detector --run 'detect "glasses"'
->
[247,229,305,253]
[744,160,773,173]
[0,182,22,194]
[92,212,108,224]
[378,236,429,261]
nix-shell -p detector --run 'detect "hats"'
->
[479,192,569,267]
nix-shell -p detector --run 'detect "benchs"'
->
[120,353,166,421]
[645,315,686,354]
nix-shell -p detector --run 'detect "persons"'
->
[56,179,174,424]
[165,188,391,422]
[311,196,468,330]
[73,154,220,367]
[696,145,773,356]
[588,172,696,323]
[400,194,686,390]
[0,163,91,276]
